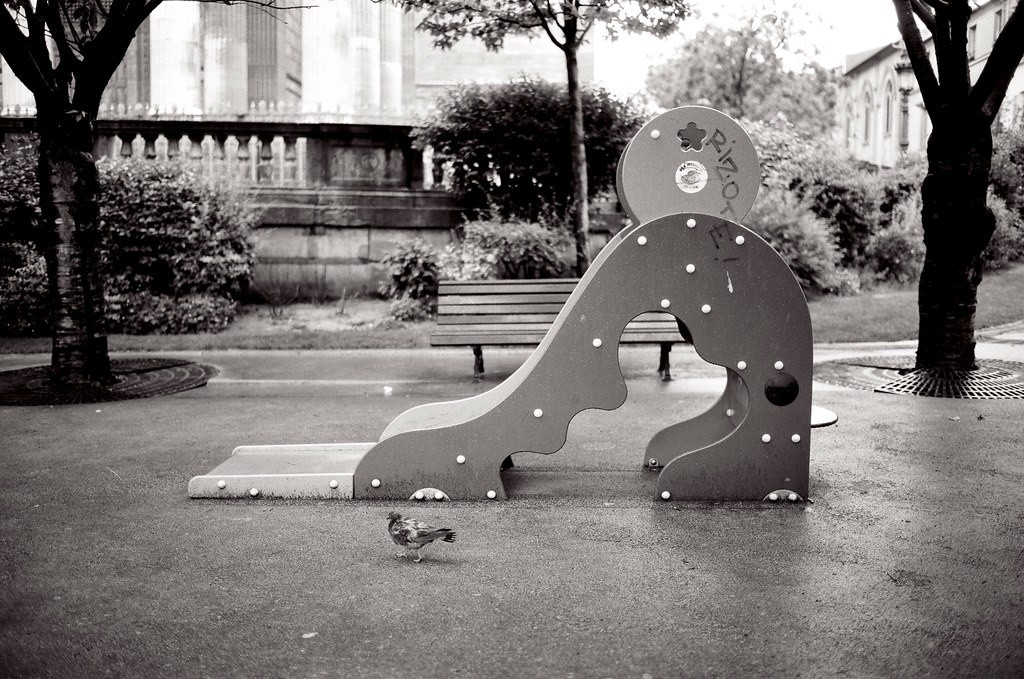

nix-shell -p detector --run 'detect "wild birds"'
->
[386,510,457,564]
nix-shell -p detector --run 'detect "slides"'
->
[185,105,840,505]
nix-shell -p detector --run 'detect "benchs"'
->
[431,278,686,382]
